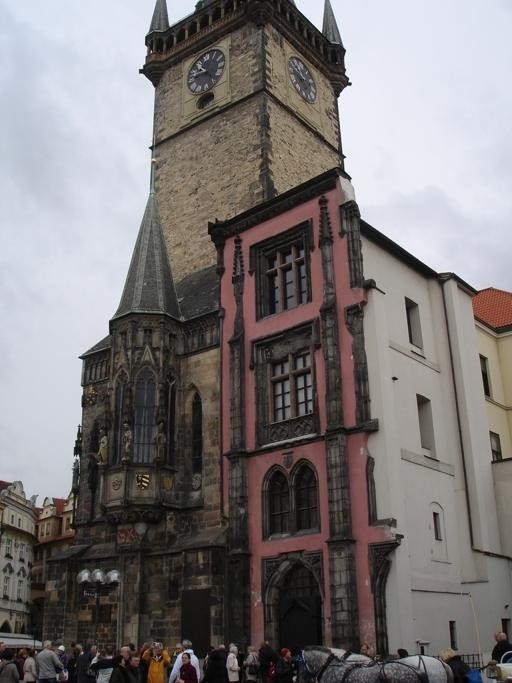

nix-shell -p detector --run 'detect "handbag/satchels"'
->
[248,654,258,674]
[464,667,483,682]
[267,663,277,678]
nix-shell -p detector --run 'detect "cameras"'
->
[152,642,160,648]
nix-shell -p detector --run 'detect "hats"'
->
[56,644,65,651]
[281,647,288,657]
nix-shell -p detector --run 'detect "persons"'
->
[487,631,511,666]
[395,647,408,658]
[437,648,472,682]
[360,640,370,656]
[1,635,297,682]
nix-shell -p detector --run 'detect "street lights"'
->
[77,567,121,649]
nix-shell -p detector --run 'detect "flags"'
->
[53,497,65,512]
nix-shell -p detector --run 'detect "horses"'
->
[296,645,430,683]
[326,647,454,683]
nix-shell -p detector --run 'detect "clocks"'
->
[287,56,319,104]
[185,46,227,95]
